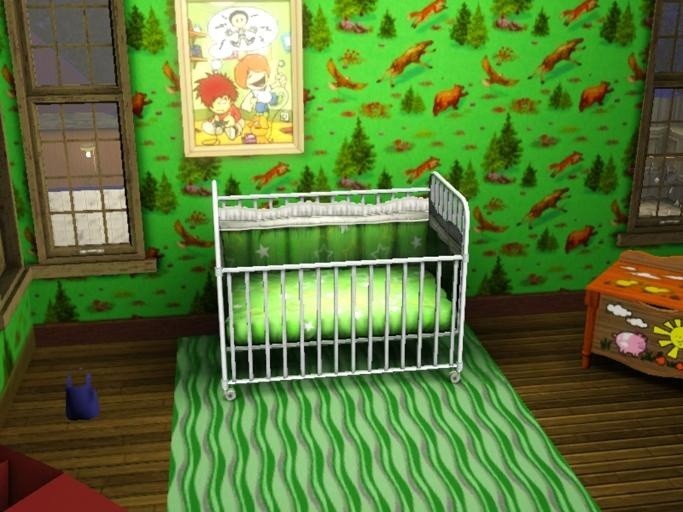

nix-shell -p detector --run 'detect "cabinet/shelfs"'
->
[579,250,683,385]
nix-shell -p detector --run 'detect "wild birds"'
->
[480,54,518,87]
[472,205,508,234]
[326,58,367,91]
[610,199,627,226]
[161,59,180,95]
[627,52,645,83]
[172,217,212,250]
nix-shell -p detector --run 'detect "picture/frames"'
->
[173,0,306,160]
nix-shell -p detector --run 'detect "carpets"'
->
[169,332,602,511]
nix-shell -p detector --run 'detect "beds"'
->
[210,171,471,402]
